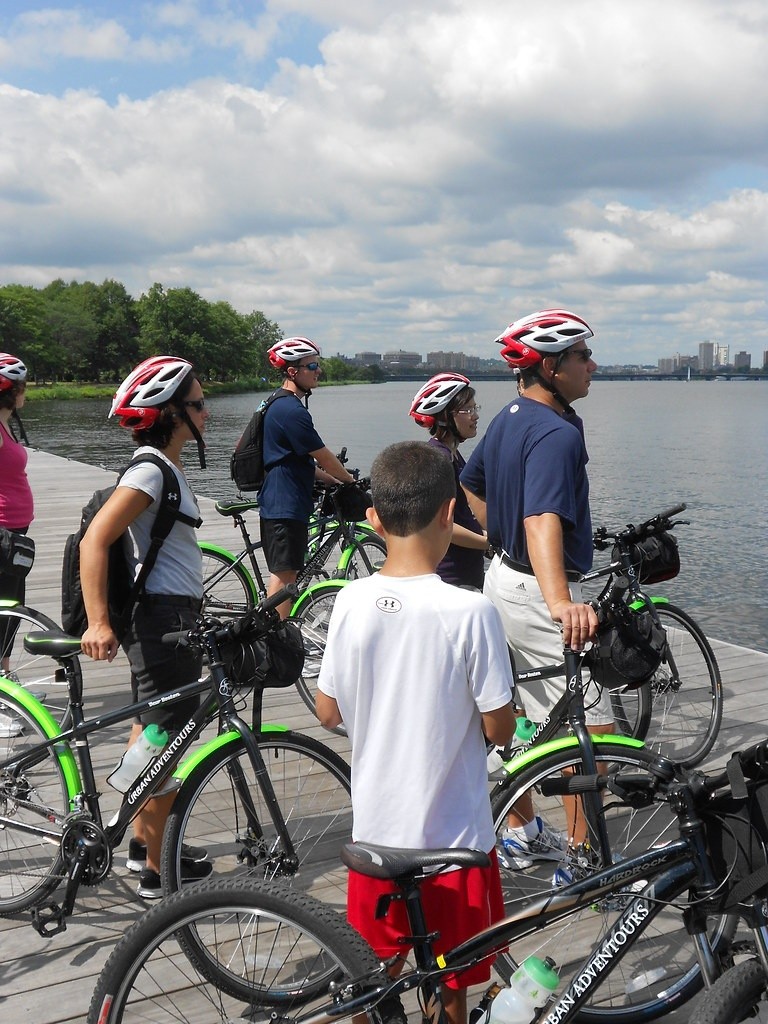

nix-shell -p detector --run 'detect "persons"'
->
[80,356,213,898]
[409,373,489,595]
[315,440,517,1024]
[257,337,357,677]
[0,353,47,737]
[459,310,647,913]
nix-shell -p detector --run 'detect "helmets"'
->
[409,372,470,428]
[0,353,28,392]
[266,337,319,369]
[495,309,593,367]
[108,355,193,430]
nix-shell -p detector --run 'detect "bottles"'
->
[109,724,169,791]
[484,717,535,773]
[472,955,559,1024]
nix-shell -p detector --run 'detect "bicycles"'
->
[87,739,768,1024]
[0,448,739,1024]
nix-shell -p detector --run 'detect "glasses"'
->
[547,349,592,357]
[292,361,319,369]
[450,404,481,417]
[180,398,205,411]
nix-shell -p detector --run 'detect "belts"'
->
[495,548,582,581]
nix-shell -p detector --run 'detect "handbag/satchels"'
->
[0,530,35,577]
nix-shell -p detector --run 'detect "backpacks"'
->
[61,455,181,635]
[230,390,292,492]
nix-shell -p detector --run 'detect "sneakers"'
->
[554,852,626,889]
[125,839,208,871]
[498,818,571,869]
[137,860,212,897]
